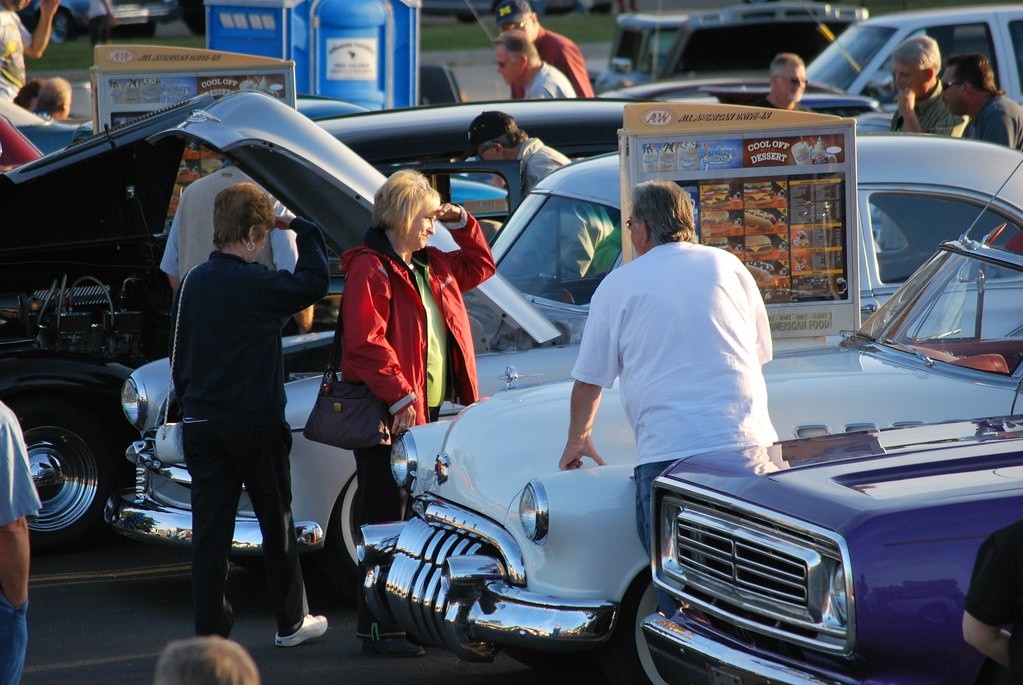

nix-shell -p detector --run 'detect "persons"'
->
[559,180,782,620]
[0,0,119,120]
[747,54,811,110]
[0,401,43,685]
[488,29,585,188]
[875,36,970,249]
[942,52,1023,149]
[171,184,331,646]
[161,158,299,356]
[469,111,614,280]
[342,171,496,656]
[495,0,595,99]
[963,520,1023,685]
[154,635,261,685]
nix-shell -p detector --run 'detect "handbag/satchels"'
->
[304,370,387,450]
[155,422,184,463]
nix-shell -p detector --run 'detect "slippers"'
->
[275,614,328,647]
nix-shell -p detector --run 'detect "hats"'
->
[496,0,531,27]
[460,111,512,162]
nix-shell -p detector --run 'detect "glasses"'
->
[941,81,960,91]
[626,220,637,230]
[475,146,490,160]
[778,77,808,85]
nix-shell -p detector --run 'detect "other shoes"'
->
[355,612,426,659]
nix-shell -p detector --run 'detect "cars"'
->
[641,406,1023,685]
[0,97,882,540]
[592,0,1023,136]
[18,0,207,44]
[351,235,1023,672]
[102,131,1023,571]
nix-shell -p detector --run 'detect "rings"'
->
[399,423,405,427]
